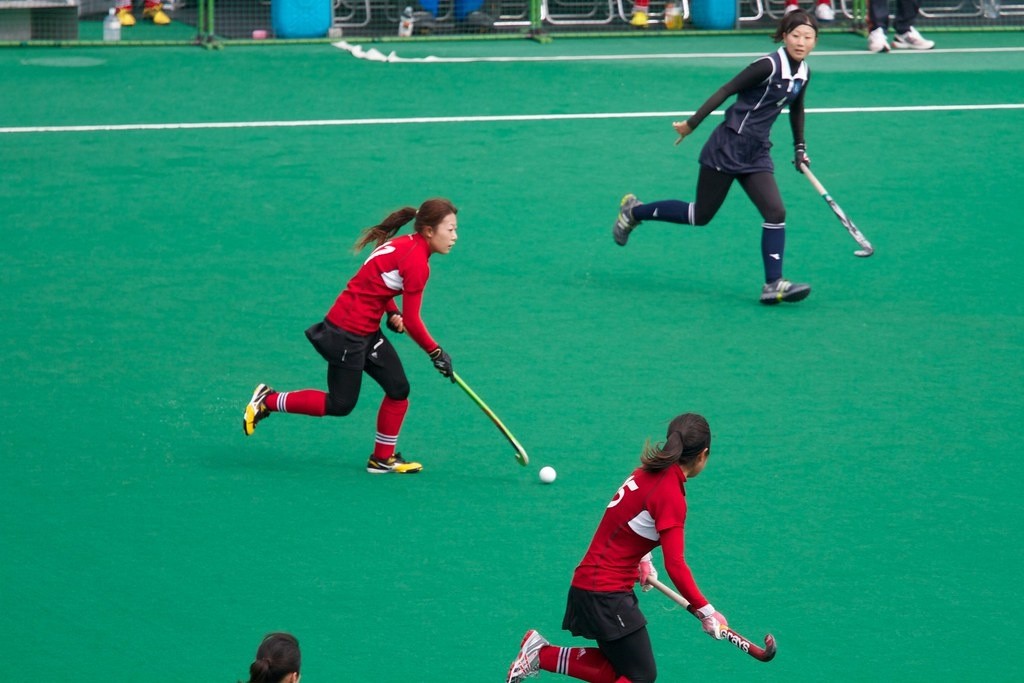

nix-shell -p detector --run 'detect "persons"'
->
[631,0,678,29]
[413,0,494,33]
[613,9,818,304]
[242,198,459,477]
[783,0,835,22]
[248,632,301,683]
[507,413,728,682]
[115,0,171,26]
[867,0,935,53]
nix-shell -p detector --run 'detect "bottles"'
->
[102,7,121,41]
[398,6,414,37]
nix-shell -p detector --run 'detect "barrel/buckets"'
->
[690,0,735,30]
[269,0,332,40]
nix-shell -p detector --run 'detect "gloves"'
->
[427,347,456,384]
[793,144,809,174]
[687,603,730,640]
[635,552,658,592]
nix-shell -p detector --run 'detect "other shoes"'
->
[461,11,493,28]
[410,10,435,30]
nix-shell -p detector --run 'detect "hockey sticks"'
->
[800,161,875,258]
[452,371,529,467]
[640,574,778,664]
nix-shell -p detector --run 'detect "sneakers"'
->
[366,455,422,476]
[612,193,641,246]
[140,4,171,25]
[116,8,136,26]
[867,28,890,54]
[891,28,935,50]
[816,3,836,21]
[761,278,809,304]
[507,628,550,683]
[784,4,798,15]
[243,384,277,435]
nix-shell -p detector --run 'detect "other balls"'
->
[539,466,557,483]
